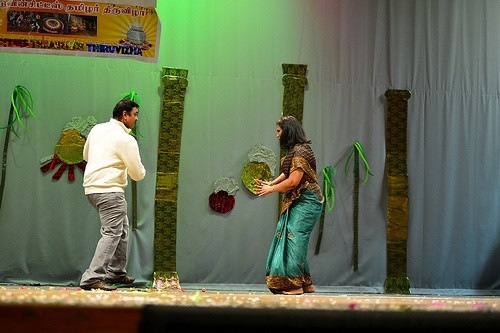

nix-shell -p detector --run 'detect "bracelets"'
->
[269,181,273,186]
[272,185,276,193]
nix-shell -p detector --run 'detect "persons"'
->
[79,101,146,292]
[254,116,324,295]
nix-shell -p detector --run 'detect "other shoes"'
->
[107,276,135,284]
[81,281,117,291]
